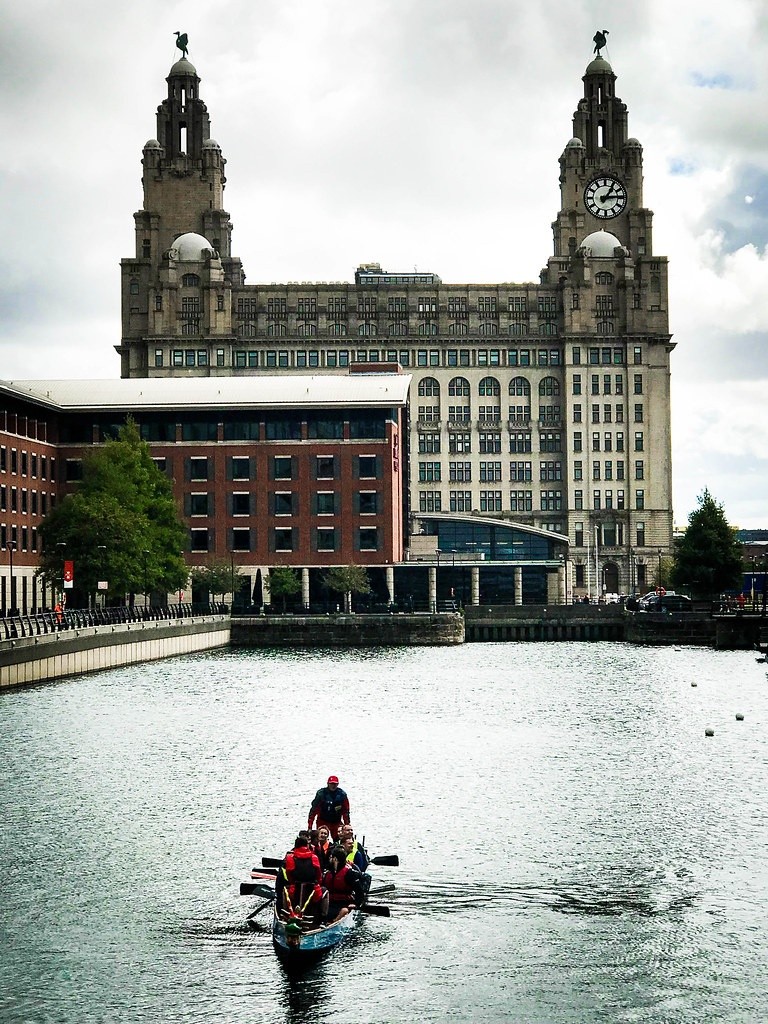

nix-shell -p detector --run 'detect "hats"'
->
[327,776,339,784]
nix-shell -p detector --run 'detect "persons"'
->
[54,590,67,623]
[308,776,351,840]
[721,594,745,613]
[575,593,588,604]
[282,824,371,929]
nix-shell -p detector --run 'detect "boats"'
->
[272,835,374,952]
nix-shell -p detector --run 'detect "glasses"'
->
[328,856,334,860]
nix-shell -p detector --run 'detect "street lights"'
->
[434,549,442,613]
[97,545,107,608]
[599,568,608,596]
[56,542,67,618]
[451,549,458,567]
[141,549,150,616]
[6,540,18,616]
[229,550,235,614]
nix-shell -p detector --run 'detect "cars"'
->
[594,590,693,613]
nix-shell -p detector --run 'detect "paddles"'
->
[367,883,395,897]
[251,867,280,882]
[368,853,401,866]
[345,899,391,918]
[238,882,279,922]
[261,855,283,869]
